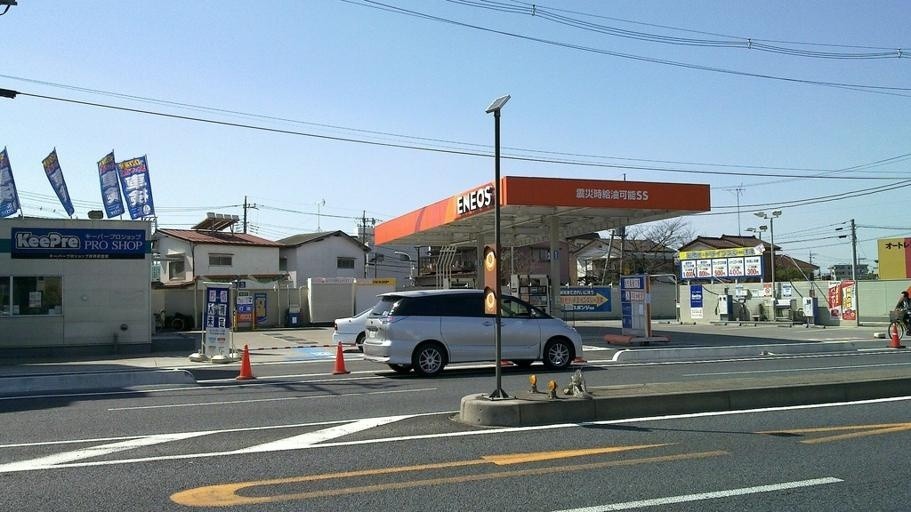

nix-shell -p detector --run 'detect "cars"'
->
[334,289,584,379]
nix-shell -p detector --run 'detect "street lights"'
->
[745,225,767,240]
[753,209,783,299]
[394,251,414,286]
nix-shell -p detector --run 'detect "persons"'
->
[898,291,911,334]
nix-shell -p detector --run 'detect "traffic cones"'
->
[235,344,257,381]
[331,340,349,375]
[886,322,906,349]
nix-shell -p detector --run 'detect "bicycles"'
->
[888,310,911,339]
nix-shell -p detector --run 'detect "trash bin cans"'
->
[289,313,301,327]
